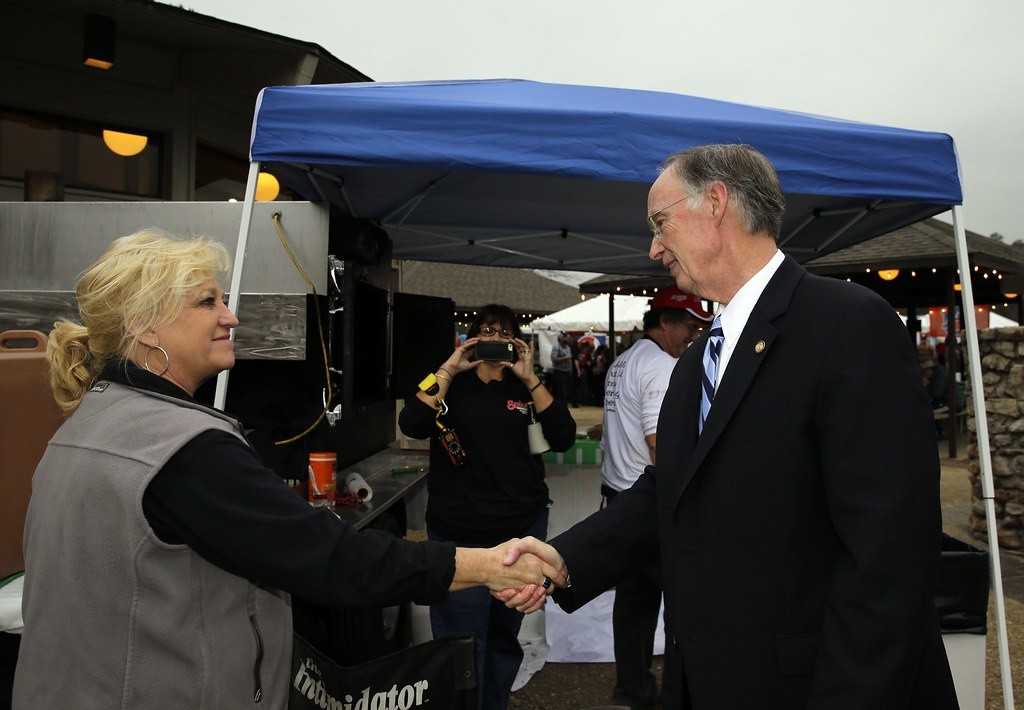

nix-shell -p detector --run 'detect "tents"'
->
[524,289,721,352]
[196,62,1021,709]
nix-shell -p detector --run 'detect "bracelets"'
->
[433,373,452,385]
[438,366,454,380]
[528,381,542,393]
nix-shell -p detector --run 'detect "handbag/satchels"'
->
[289,634,478,710]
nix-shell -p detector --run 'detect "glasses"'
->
[478,325,514,340]
[646,189,705,235]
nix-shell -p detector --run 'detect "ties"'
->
[696,313,726,435]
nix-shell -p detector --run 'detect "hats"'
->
[646,283,714,324]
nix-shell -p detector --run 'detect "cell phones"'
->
[475,341,513,362]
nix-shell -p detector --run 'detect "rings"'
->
[543,577,552,591]
[520,350,525,354]
[510,363,513,368]
[518,352,521,355]
[523,350,529,354]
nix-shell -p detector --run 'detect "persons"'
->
[492,140,964,710]
[7,228,570,710]
[910,325,977,421]
[546,333,619,402]
[395,297,579,709]
[599,284,715,704]
